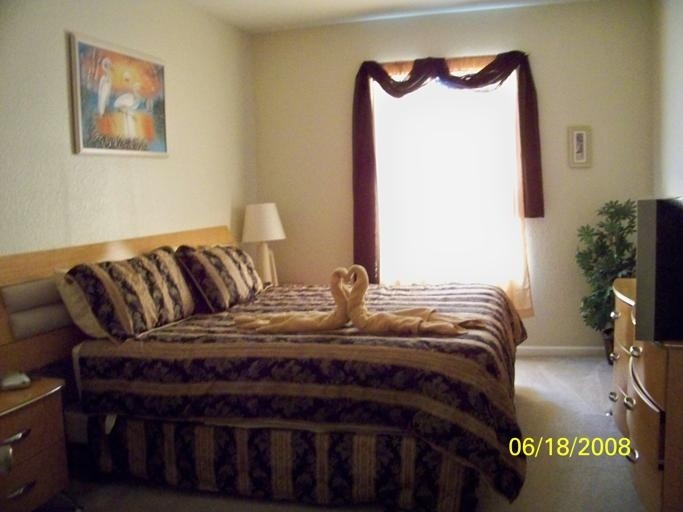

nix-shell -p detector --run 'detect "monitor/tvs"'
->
[634,194,683,342]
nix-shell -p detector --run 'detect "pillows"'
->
[52,238,269,344]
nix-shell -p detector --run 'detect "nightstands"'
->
[0,374,71,511]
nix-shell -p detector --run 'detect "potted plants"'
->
[573,198,637,364]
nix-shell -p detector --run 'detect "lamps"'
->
[239,201,288,283]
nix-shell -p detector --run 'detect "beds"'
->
[0,223,527,510]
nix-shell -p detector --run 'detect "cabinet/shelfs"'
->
[607,276,681,512]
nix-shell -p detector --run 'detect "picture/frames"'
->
[68,29,170,160]
[567,126,592,167]
